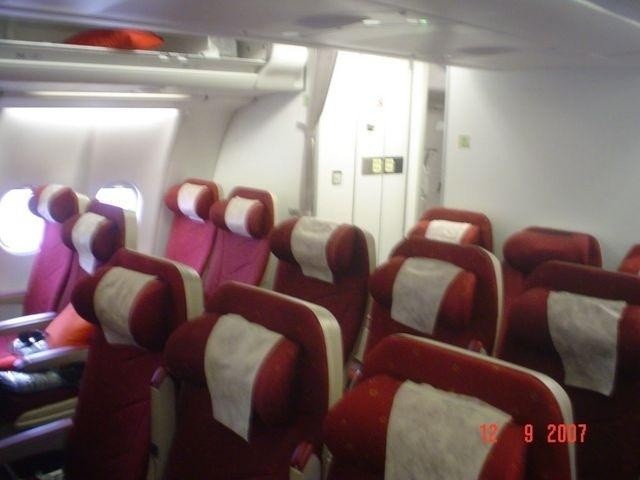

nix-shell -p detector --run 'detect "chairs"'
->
[2,179,80,365]
[491,256,639,431]
[152,276,348,480]
[1,245,205,480]
[263,214,380,363]
[337,234,506,397]
[198,182,277,312]
[501,222,605,292]
[0,198,141,461]
[158,179,223,282]
[401,205,495,257]
[288,330,582,480]
